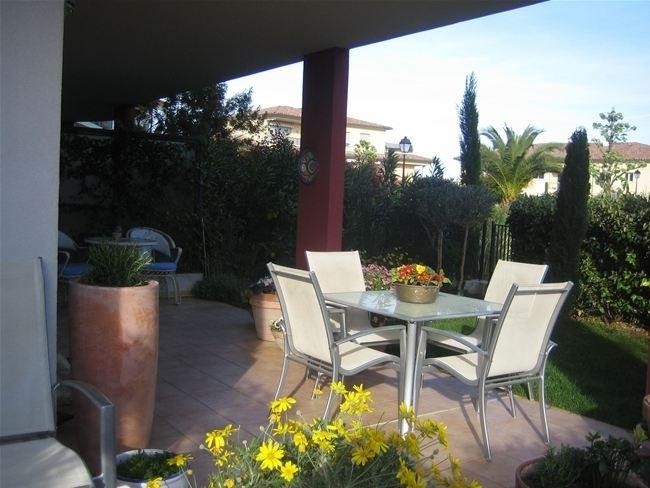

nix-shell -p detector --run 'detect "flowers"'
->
[252,275,276,293]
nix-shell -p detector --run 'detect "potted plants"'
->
[116,449,187,488]
[515,433,600,488]
[66,238,160,448]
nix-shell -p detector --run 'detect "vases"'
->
[249,291,282,341]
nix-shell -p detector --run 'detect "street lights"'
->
[400,136,411,185]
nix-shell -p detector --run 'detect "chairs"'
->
[58,227,182,306]
[0,256,116,488]
